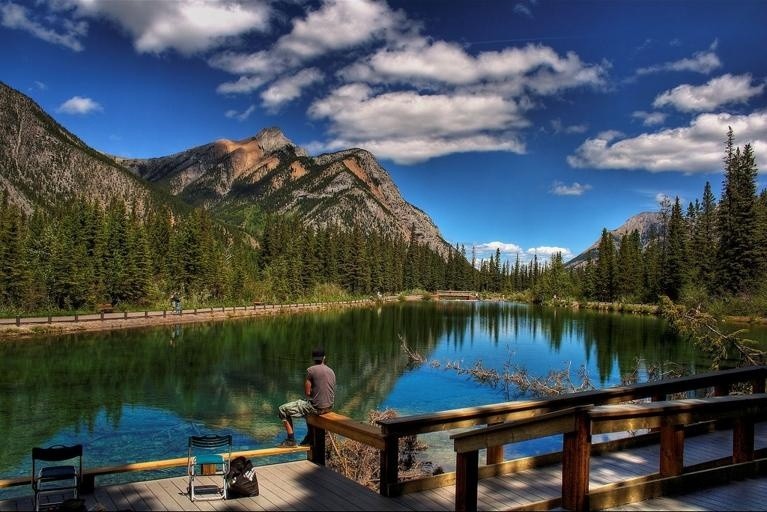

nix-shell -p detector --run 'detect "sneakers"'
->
[275,436,313,448]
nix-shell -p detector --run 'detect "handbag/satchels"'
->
[225,456,259,500]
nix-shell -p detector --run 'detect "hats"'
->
[312,348,324,360]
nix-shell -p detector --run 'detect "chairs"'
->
[33,445,83,511]
[188,434,233,501]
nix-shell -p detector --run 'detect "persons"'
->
[169,325,180,348]
[170,292,180,314]
[275,348,336,448]
